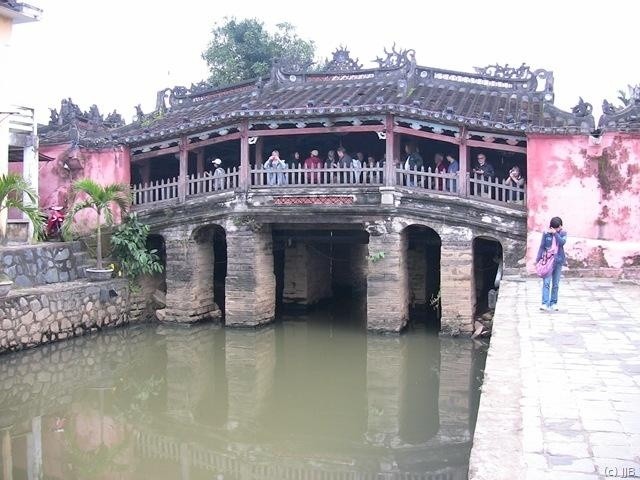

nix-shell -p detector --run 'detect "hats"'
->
[212,158,221,165]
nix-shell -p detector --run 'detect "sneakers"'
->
[539,304,548,310]
[551,304,559,311]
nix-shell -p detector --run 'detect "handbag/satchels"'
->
[533,236,558,279]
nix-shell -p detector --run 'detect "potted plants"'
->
[0,172,51,300]
[57,178,137,283]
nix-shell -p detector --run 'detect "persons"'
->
[211,157,226,190]
[288,150,305,184]
[532,216,568,311]
[324,142,459,193]
[472,153,496,200]
[504,166,527,202]
[303,148,323,184]
[263,149,289,186]
